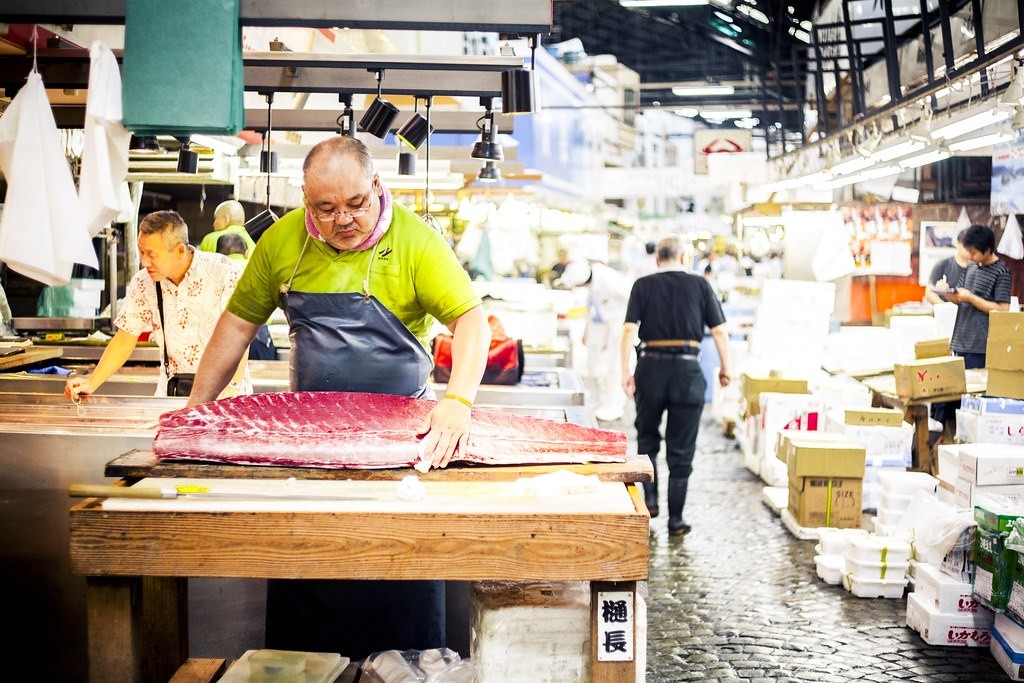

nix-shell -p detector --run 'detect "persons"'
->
[186,136,492,468]
[925,228,976,305]
[200,201,257,259]
[697,244,785,303]
[620,238,733,536]
[946,225,1012,369]
[641,241,657,268]
[216,233,277,361]
[64,210,254,402]
[551,249,593,289]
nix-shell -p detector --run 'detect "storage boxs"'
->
[742,308,1024,683]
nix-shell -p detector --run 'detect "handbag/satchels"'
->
[166,373,195,396]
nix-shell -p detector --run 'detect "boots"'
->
[645,454,659,518]
[665,476,693,536]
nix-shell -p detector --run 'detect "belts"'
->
[644,351,696,362]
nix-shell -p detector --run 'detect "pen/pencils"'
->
[942,274,946,292]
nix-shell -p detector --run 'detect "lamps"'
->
[1001,56,1024,129]
[909,99,934,143]
[243,90,279,243]
[396,140,418,175]
[396,95,435,150]
[358,68,400,139]
[338,92,357,139]
[174,135,198,174]
[854,121,884,159]
[128,134,160,153]
[470,96,505,162]
[500,32,541,116]
[478,160,501,182]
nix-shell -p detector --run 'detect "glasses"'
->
[67,371,86,418]
[303,185,375,221]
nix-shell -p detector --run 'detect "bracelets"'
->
[444,394,473,409]
